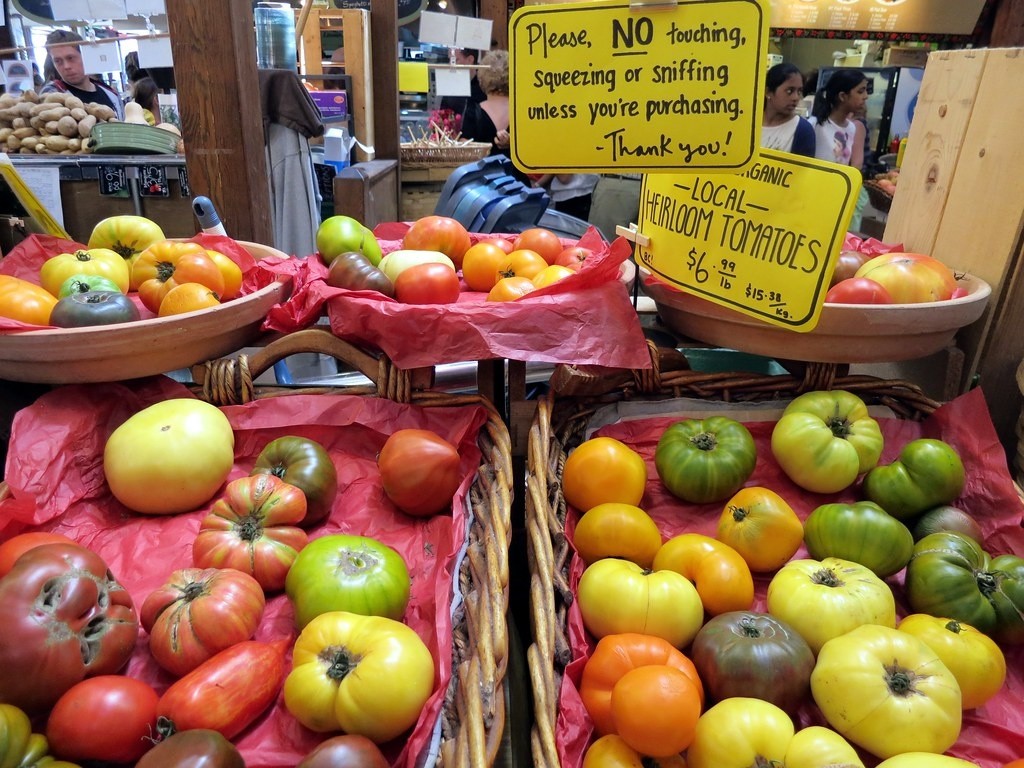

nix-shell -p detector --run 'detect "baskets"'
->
[399,140,492,163]
[526,337,1024,768]
[0,328,514,768]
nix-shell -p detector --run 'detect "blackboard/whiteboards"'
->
[314,163,336,201]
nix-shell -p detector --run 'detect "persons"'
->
[125,51,161,126]
[761,63,816,158]
[461,49,532,188]
[440,47,487,119]
[532,174,601,221]
[807,69,871,173]
[32,29,125,121]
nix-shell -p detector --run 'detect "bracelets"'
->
[535,183,540,188]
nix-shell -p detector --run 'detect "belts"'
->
[600,173,640,181]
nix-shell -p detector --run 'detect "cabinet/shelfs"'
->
[296,8,373,163]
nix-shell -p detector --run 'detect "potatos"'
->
[0,89,121,154]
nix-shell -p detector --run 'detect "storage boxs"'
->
[832,46,930,68]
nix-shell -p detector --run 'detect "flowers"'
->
[425,108,464,142]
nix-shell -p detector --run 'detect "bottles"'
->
[890,133,899,154]
[871,126,879,151]
[896,133,908,172]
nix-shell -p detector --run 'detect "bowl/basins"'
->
[87,121,180,154]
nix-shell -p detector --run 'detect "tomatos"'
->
[0,399,465,768]
[876,171,898,195]
[0,214,242,328]
[316,214,594,302]
[824,247,959,305]
[562,388,1024,768]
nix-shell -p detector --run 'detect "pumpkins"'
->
[123,98,181,136]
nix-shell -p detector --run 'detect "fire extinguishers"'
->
[890,134,901,153]
